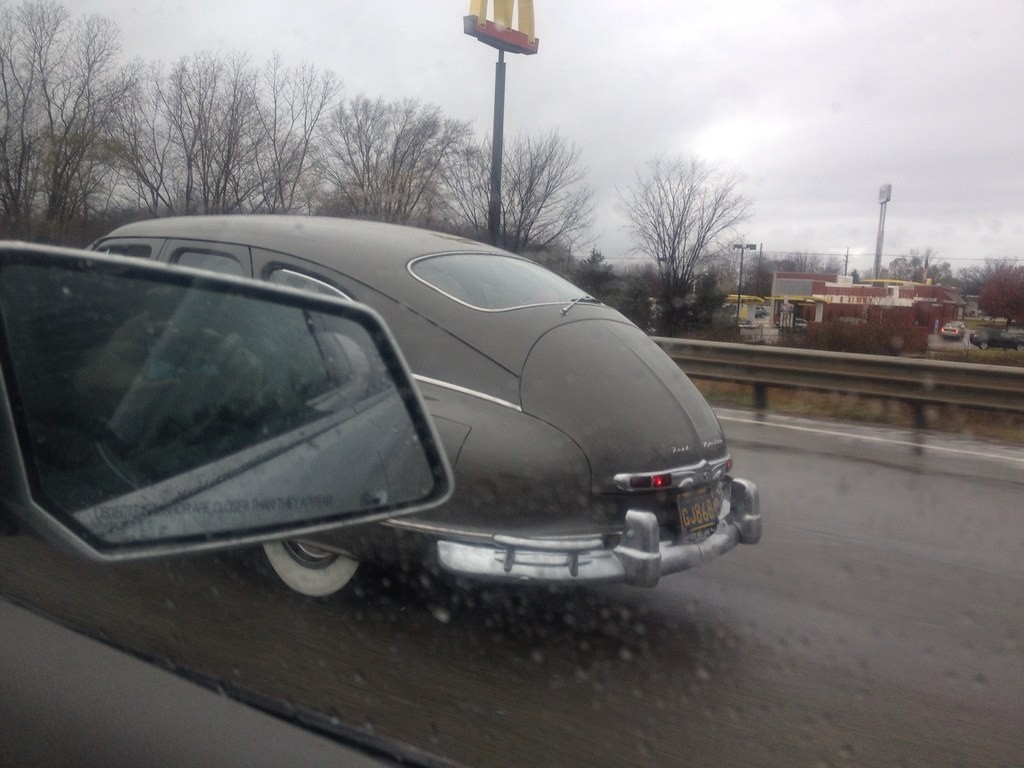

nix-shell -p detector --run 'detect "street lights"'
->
[731,242,758,328]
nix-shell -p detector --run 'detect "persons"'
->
[33,289,281,482]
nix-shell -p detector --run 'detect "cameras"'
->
[135,325,200,380]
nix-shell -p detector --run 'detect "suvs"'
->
[969,327,1024,352]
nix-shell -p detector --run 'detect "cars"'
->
[83,211,767,612]
[941,322,967,340]
[779,318,808,335]
[755,303,766,318]
